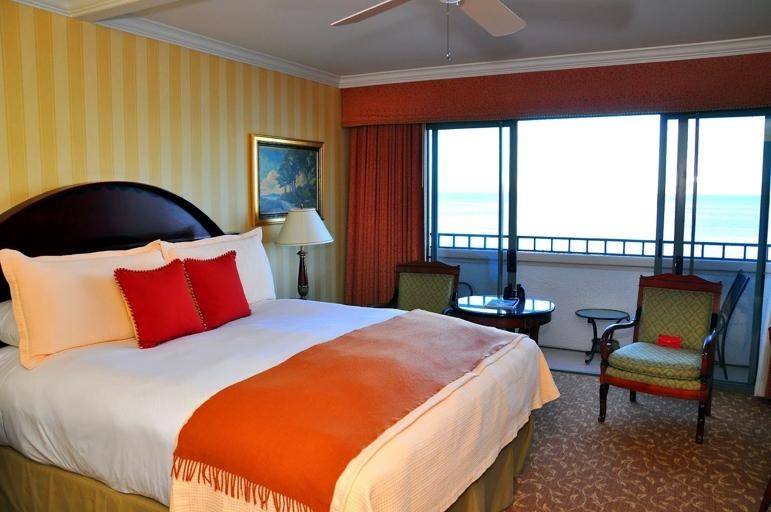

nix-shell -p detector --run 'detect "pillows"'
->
[1,240,167,372]
[158,230,276,304]
[112,259,206,348]
[181,251,253,330]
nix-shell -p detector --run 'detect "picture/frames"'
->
[248,134,328,229]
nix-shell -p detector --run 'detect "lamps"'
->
[274,204,336,299]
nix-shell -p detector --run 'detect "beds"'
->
[0,179,541,512]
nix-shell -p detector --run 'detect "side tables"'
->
[451,294,556,344]
[574,308,629,364]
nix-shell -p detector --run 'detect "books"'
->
[485,298,520,309]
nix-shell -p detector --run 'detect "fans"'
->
[327,1,528,38]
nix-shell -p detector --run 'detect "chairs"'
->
[596,268,752,445]
[365,256,460,318]
